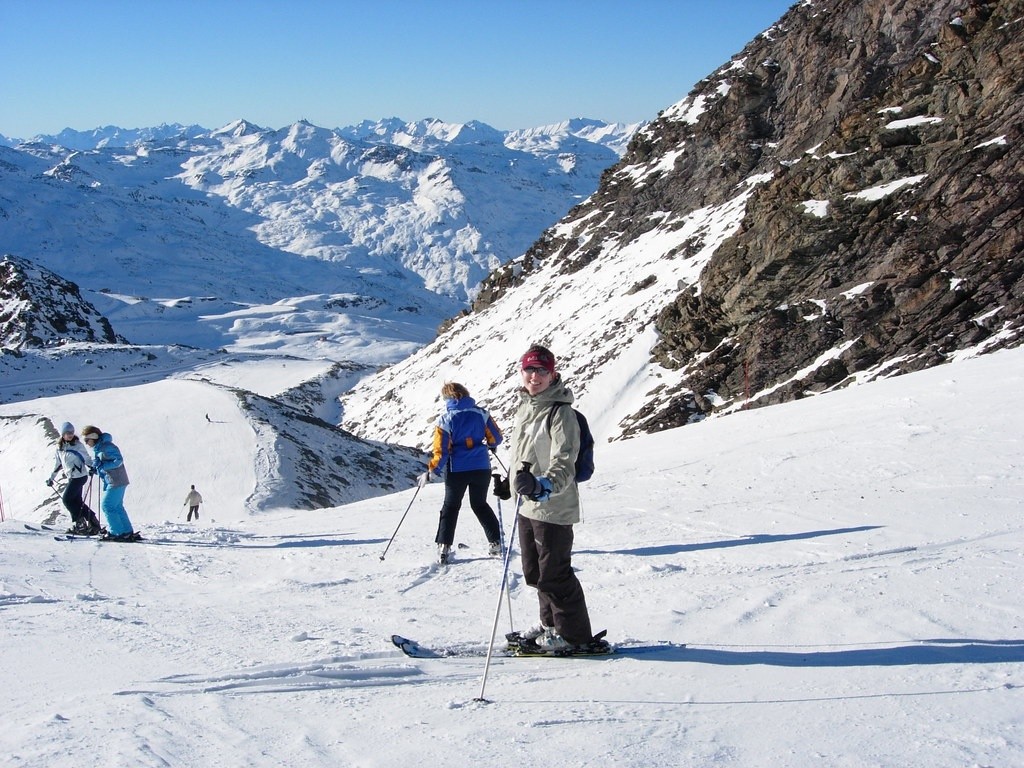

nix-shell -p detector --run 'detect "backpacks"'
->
[546,400,594,482]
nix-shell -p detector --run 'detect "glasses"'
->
[84,438,90,443]
[523,366,550,377]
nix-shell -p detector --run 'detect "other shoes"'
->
[69,524,88,533]
[105,533,130,540]
[88,523,100,533]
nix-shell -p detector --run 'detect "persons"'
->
[184,484,202,522]
[493,347,593,651]
[46,422,133,539]
[417,382,505,558]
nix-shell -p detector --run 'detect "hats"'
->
[61,421,74,436]
[520,351,555,372]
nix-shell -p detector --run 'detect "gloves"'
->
[93,459,102,468]
[494,477,513,500]
[89,467,97,476]
[514,469,543,496]
[417,471,435,487]
[46,478,54,486]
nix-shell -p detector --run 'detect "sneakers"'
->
[488,540,504,554]
[536,629,574,649]
[438,541,449,560]
[520,620,548,639]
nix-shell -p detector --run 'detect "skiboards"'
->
[391,629,612,659]
[24,524,108,536]
[53,530,150,544]
[435,543,511,567]
